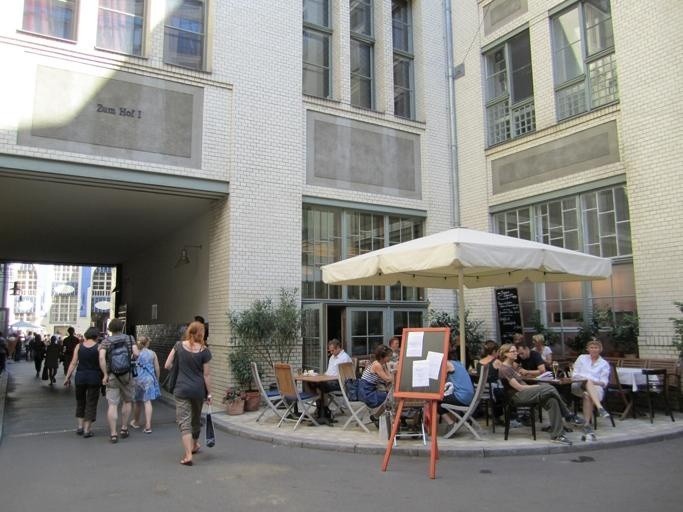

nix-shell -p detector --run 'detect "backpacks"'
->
[106,335,131,375]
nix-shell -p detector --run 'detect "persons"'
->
[387,337,400,362]
[163,321,213,466]
[194,316,209,341]
[437,352,475,439]
[359,345,395,430]
[314,339,351,419]
[98,319,137,443]
[0,328,106,386]
[448,331,552,429]
[128,336,160,433]
[497,344,584,446]
[571,341,609,440]
[63,327,106,438]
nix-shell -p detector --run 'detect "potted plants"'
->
[222,284,309,416]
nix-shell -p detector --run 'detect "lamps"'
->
[111,278,129,293]
[176,242,203,264]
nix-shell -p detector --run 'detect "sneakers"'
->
[444,408,609,444]
[370,415,378,427]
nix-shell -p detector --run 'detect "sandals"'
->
[77,420,200,466]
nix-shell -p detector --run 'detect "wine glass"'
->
[552,359,559,380]
[568,362,575,378]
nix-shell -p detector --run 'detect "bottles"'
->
[303,369,307,376]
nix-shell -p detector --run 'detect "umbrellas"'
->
[320,227,611,369]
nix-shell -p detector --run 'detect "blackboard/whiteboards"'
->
[393,327,450,401]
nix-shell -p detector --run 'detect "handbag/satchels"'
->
[42,365,48,380]
[344,378,359,400]
[160,354,178,394]
[205,405,216,447]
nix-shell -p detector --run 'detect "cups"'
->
[307,370,314,376]
